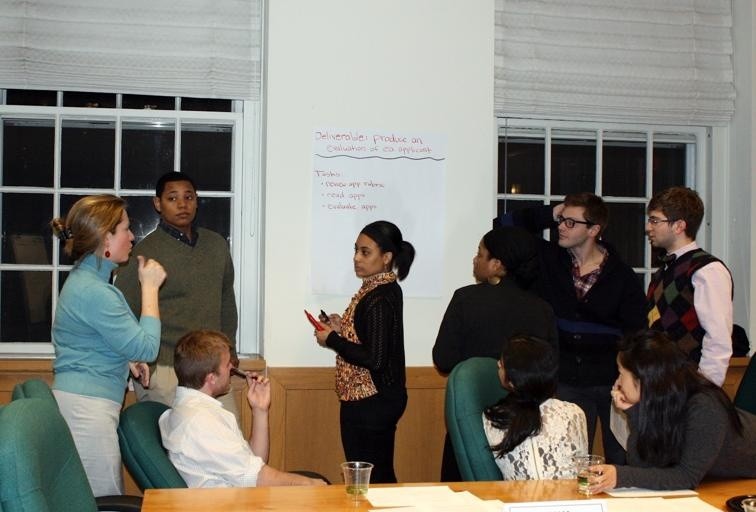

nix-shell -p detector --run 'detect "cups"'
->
[575,453,604,495]
[340,460,375,503]
[741,499,756,512]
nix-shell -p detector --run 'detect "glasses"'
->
[647,218,675,226]
[557,214,594,228]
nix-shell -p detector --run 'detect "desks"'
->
[140,475,755,512]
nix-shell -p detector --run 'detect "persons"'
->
[113,172,239,430]
[50,194,168,498]
[432,228,554,481]
[315,221,415,483]
[493,192,648,466]
[644,186,734,388]
[482,329,589,480]
[158,329,332,488]
[586,329,756,495]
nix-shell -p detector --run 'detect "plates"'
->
[726,494,756,512]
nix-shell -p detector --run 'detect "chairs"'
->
[441,356,516,480]
[118,399,188,491]
[11,380,142,511]
[0,392,101,511]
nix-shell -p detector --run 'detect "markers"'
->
[304,309,323,331]
[230,368,257,381]
[320,310,331,324]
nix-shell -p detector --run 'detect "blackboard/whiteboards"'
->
[263,0,497,367]
